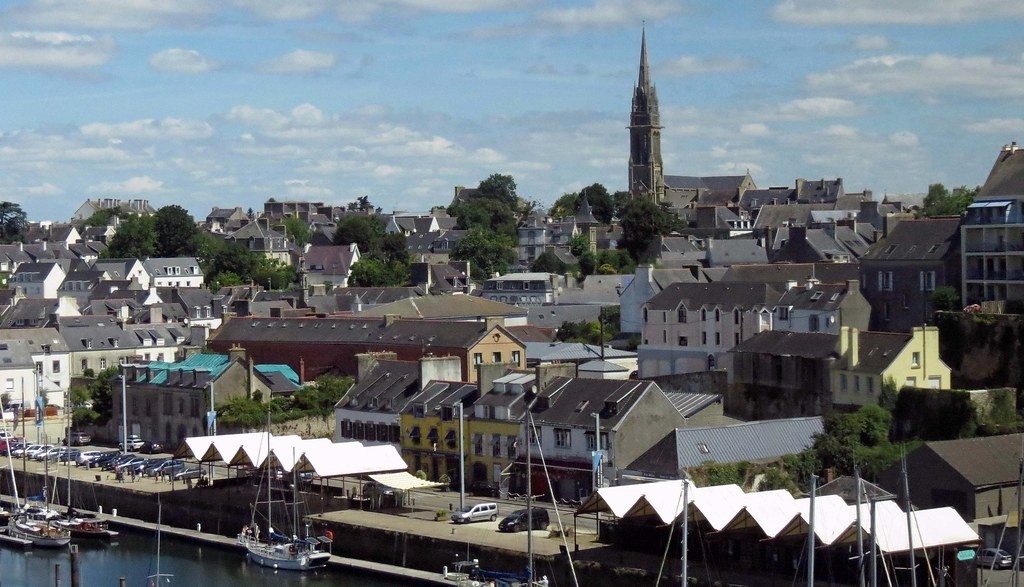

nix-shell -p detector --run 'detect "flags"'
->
[35,395,45,428]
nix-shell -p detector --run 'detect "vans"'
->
[498,508,550,532]
[450,502,500,525]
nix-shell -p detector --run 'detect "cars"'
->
[0,431,159,476]
[169,467,207,481]
[139,441,165,455]
[148,458,185,478]
[363,482,402,502]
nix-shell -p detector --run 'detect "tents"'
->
[170,432,450,514]
[572,478,985,585]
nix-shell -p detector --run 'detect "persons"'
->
[81,522,109,531]
[537,575,549,586]
[112,464,209,488]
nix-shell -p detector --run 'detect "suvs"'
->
[117,432,145,452]
[61,432,92,446]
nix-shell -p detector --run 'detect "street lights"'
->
[588,410,601,481]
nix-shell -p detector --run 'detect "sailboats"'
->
[234,396,336,571]
[0,400,75,547]
[18,363,120,539]
